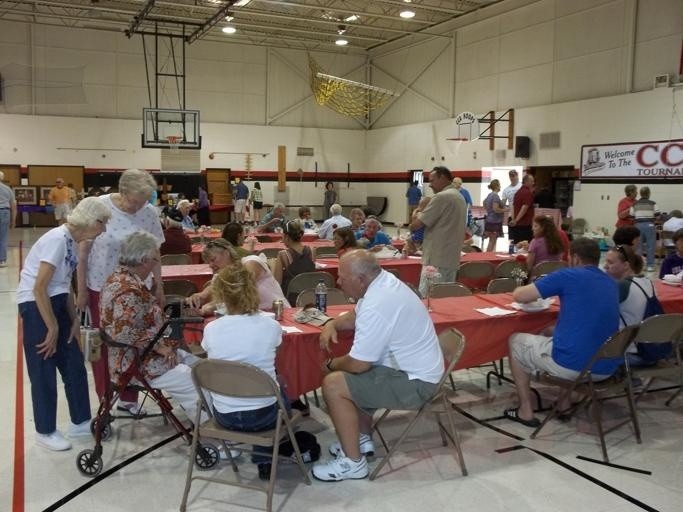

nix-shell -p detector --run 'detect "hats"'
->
[294,305,336,328]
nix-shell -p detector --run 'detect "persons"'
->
[0,171,16,268]
[504,214,672,428]
[18,197,113,451]
[167,186,210,230]
[257,204,392,249]
[406,167,472,299]
[325,182,338,220]
[77,168,165,415]
[308,249,445,481]
[98,230,210,426]
[46,178,104,226]
[615,185,683,280]
[483,169,558,253]
[186,238,321,480]
[232,175,263,227]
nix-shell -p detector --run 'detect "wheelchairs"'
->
[74,270,219,478]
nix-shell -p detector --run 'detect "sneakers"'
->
[115,399,148,417]
[34,428,72,451]
[206,444,242,460]
[68,417,97,438]
[0,260,7,268]
[328,431,375,458]
[310,446,370,482]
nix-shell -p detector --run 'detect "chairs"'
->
[529,324,642,458]
[81,167,679,413]
[179,360,313,509]
[623,312,681,412]
[364,326,467,481]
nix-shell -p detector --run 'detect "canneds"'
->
[272,300,283,321]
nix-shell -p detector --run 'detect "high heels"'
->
[256,461,274,481]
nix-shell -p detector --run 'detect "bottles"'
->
[508,240,514,253]
[314,280,326,312]
[517,240,529,252]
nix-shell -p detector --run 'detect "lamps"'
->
[397,0,416,20]
[335,25,347,46]
[221,12,237,35]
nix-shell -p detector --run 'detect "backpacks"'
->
[617,278,673,362]
[280,245,316,299]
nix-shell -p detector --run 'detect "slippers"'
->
[548,399,573,422]
[502,407,542,428]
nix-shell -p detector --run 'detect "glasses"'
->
[205,241,233,258]
[55,181,61,183]
[615,244,633,265]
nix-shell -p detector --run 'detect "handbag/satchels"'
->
[70,304,103,364]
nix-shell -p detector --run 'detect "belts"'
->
[0,207,10,210]
